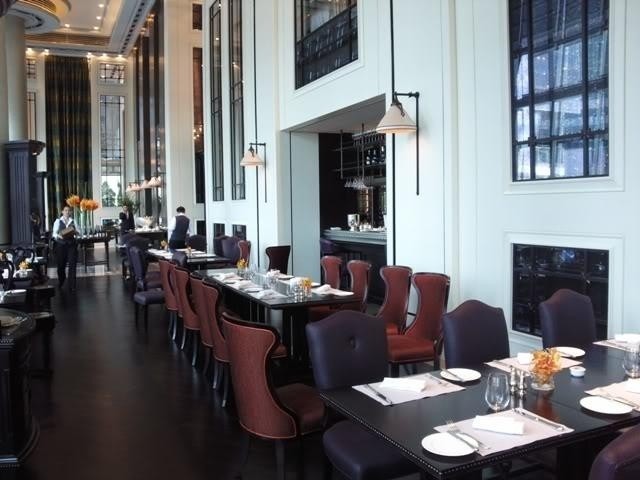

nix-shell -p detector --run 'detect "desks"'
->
[74,233,117,272]
[194,266,363,342]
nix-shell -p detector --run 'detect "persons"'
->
[51,205,80,293]
[167,206,190,250]
[117,204,135,237]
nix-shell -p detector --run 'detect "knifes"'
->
[446,431,478,451]
[439,366,464,383]
[513,406,564,431]
[366,383,393,405]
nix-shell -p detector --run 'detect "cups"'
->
[351,224,372,231]
[485,375,510,412]
[238,264,312,300]
[569,366,586,378]
[621,343,640,377]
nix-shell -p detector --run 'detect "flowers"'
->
[67,193,100,211]
[528,346,560,381]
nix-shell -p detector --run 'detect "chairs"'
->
[30,208,56,275]
[375,266,413,333]
[439,297,511,370]
[387,272,451,377]
[100,208,291,365]
[346,259,372,314]
[304,310,426,479]
[202,281,288,407]
[221,311,327,479]
[538,286,598,351]
[587,420,640,480]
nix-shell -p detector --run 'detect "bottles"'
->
[510,363,528,397]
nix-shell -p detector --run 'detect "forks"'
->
[446,419,490,449]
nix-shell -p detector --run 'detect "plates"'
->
[440,367,482,383]
[579,396,633,414]
[553,345,584,358]
[421,433,479,458]
[6,289,26,294]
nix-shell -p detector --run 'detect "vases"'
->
[528,374,557,392]
[73,206,94,230]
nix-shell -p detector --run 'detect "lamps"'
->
[125,172,165,208]
[240,141,268,203]
[374,87,421,196]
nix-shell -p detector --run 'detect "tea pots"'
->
[347,214,360,227]
